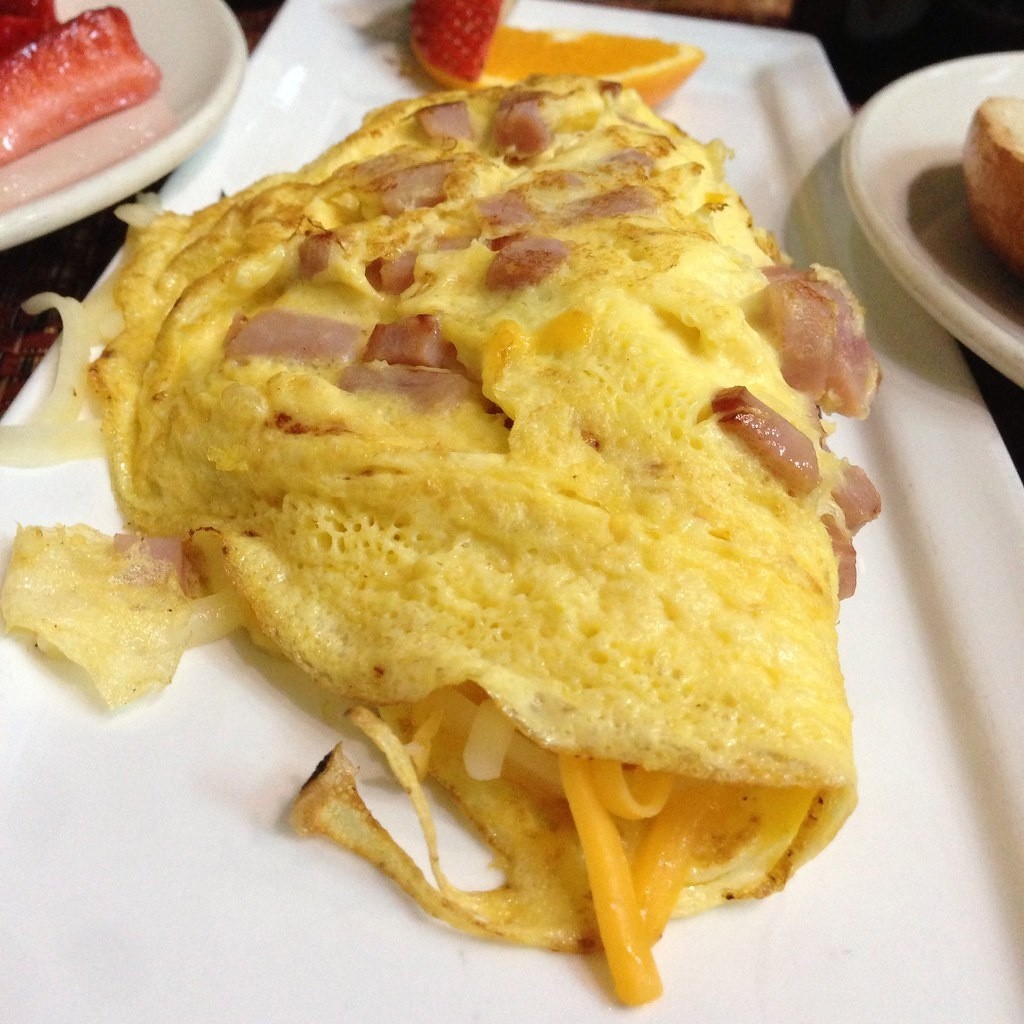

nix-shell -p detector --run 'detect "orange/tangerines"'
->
[408,26,706,108]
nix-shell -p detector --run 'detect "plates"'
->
[3,0,1024,1024]
[0,0,248,254]
[843,51,1024,392]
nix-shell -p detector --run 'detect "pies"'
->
[89,70,870,951]
